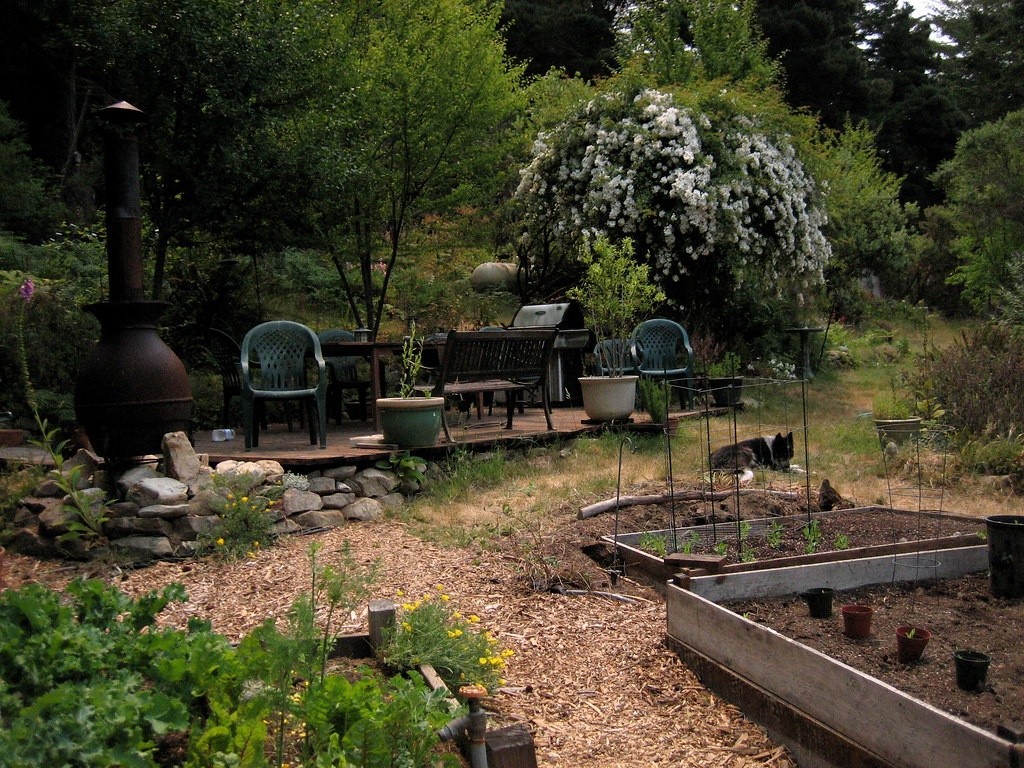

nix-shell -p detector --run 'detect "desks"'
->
[280,328,589,431]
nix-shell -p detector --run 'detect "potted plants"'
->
[680,331,728,395]
[565,230,667,421]
[872,388,923,448]
[373,321,444,448]
[986,514,1024,601]
[706,350,745,407]
[635,372,678,436]
[895,626,932,665]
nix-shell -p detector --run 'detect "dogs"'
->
[709,432,817,485]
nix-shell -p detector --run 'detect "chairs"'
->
[631,319,696,413]
[319,328,373,427]
[240,320,328,450]
[314,327,373,427]
[591,338,638,379]
[205,327,295,436]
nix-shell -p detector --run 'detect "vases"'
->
[953,651,991,690]
[804,587,834,618]
[840,605,874,638]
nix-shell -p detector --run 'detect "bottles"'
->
[212,428,235,442]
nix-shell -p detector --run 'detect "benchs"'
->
[409,327,559,441]
